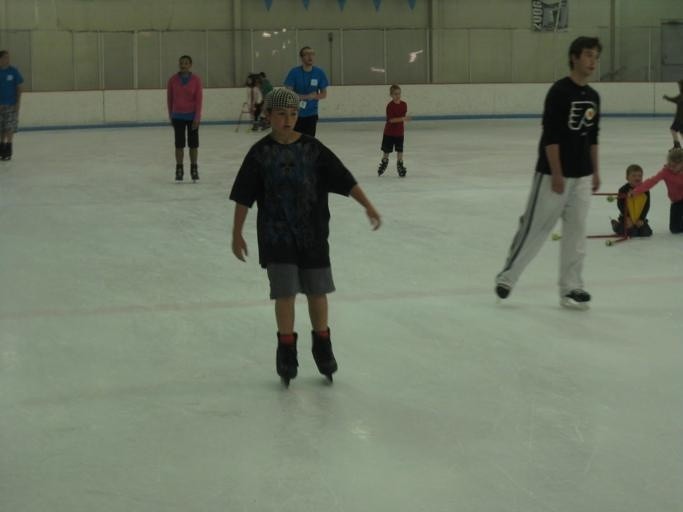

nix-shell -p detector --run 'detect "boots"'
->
[175,164,184,180]
[191,164,199,180]
[311,326,338,375]
[276,332,299,378]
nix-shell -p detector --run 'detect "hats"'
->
[266,87,299,109]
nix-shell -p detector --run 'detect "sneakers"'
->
[378,158,389,174]
[565,289,591,302]
[496,285,512,298]
[397,160,406,174]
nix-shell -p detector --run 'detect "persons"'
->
[227,87,382,380]
[611,163,652,237]
[283,45,329,138]
[0,49,24,161]
[662,80,683,149]
[630,147,683,234]
[495,35,604,303]
[166,54,203,181]
[377,83,409,175]
[244,71,273,130]
